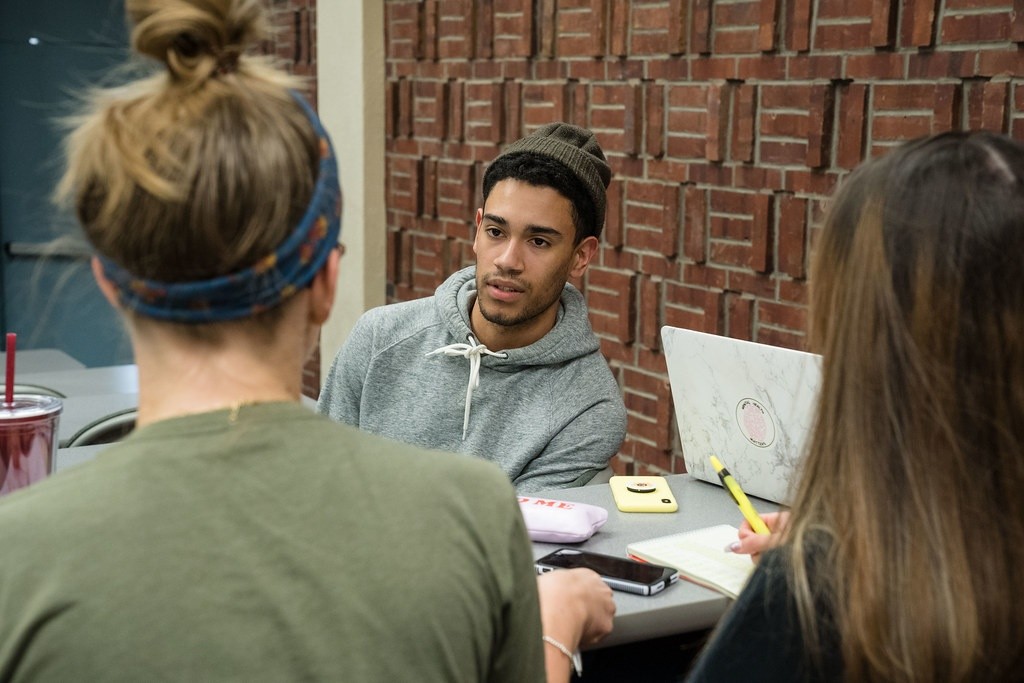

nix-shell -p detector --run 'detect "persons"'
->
[1,0,617,683]
[687,129,1023,683]
[315,122,627,493]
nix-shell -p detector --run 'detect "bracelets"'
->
[542,636,582,677]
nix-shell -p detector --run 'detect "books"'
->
[627,524,755,600]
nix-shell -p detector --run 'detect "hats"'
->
[481,122,612,237]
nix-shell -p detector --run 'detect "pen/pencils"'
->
[707,453,772,535]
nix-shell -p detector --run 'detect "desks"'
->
[522,473,795,652]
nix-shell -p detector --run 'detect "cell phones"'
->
[533,547,679,596]
[608,475,678,513]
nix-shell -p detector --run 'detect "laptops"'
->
[660,325,824,508]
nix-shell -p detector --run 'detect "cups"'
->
[0,382,68,494]
